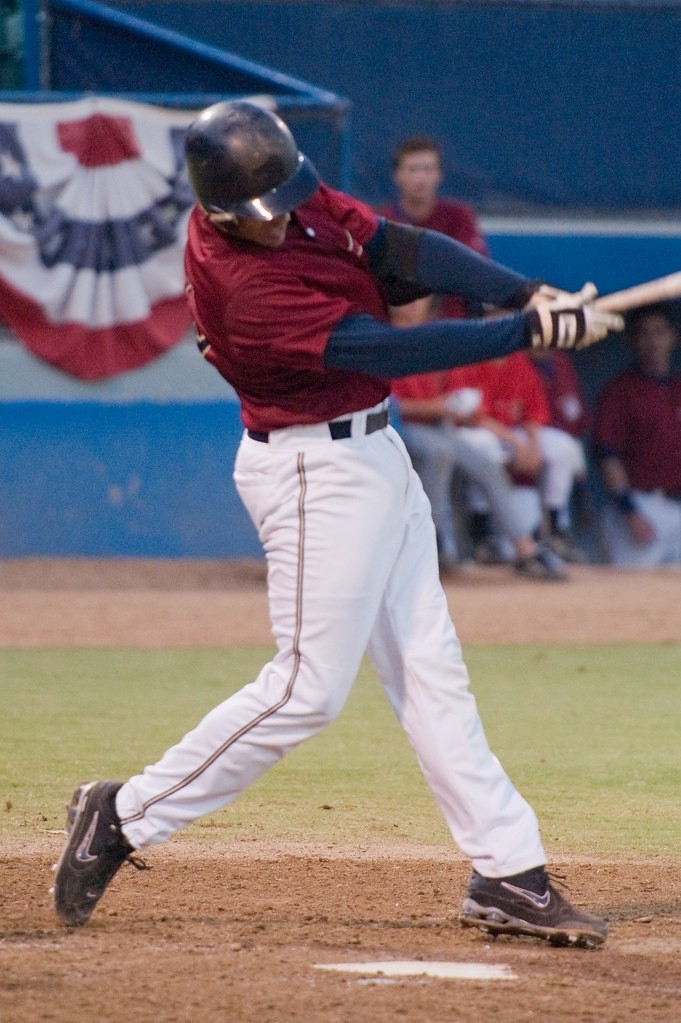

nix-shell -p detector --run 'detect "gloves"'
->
[502,281,625,353]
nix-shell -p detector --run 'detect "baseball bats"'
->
[588,270,681,315]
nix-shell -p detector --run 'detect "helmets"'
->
[184,101,320,235]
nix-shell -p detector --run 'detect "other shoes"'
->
[538,521,589,561]
[515,543,573,581]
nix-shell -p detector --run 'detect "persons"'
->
[383,281,655,582]
[47,100,626,949]
[587,307,681,586]
[367,135,503,321]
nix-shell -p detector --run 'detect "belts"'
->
[248,403,391,444]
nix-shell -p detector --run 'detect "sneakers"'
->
[48,781,155,927]
[460,870,609,948]
